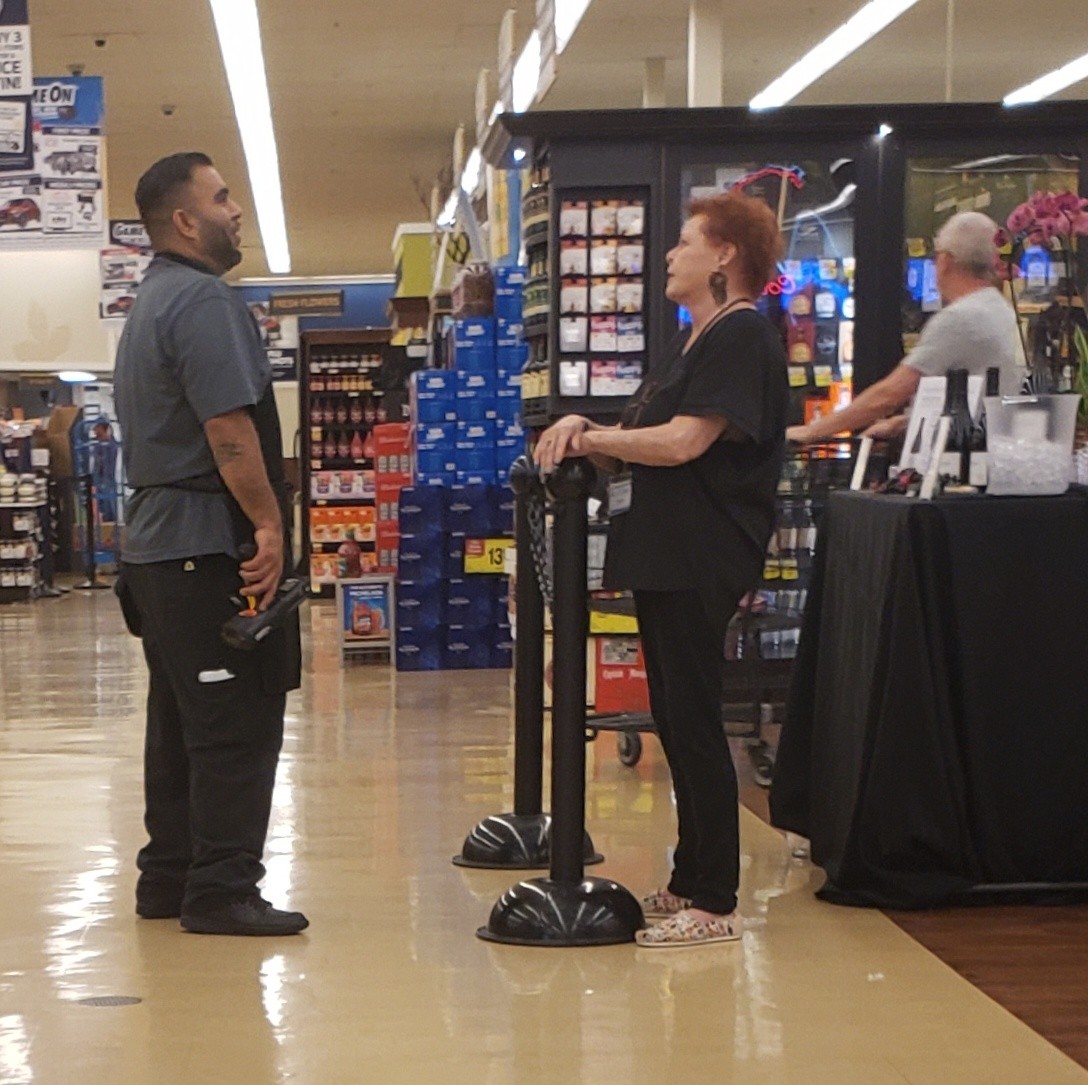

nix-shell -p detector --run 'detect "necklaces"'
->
[628,297,755,427]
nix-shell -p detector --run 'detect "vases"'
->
[983,393,1081,494]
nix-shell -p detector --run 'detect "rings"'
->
[545,439,553,448]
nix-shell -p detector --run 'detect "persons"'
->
[113,151,310,936]
[92,417,118,521]
[785,210,1027,444]
[533,192,790,948]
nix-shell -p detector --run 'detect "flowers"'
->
[994,189,1088,392]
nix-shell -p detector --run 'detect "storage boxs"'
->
[375,266,530,671]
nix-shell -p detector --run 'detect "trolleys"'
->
[585,434,907,792]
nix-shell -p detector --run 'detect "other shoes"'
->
[180,893,311,936]
[638,887,692,919]
[136,894,180,919]
[635,909,744,947]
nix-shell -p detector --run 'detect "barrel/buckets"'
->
[985,393,1081,492]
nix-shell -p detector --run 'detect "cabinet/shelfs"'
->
[0,417,58,601]
[519,134,1088,674]
[300,327,393,597]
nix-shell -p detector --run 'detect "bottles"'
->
[906,417,926,467]
[968,366,1001,492]
[931,367,975,496]
